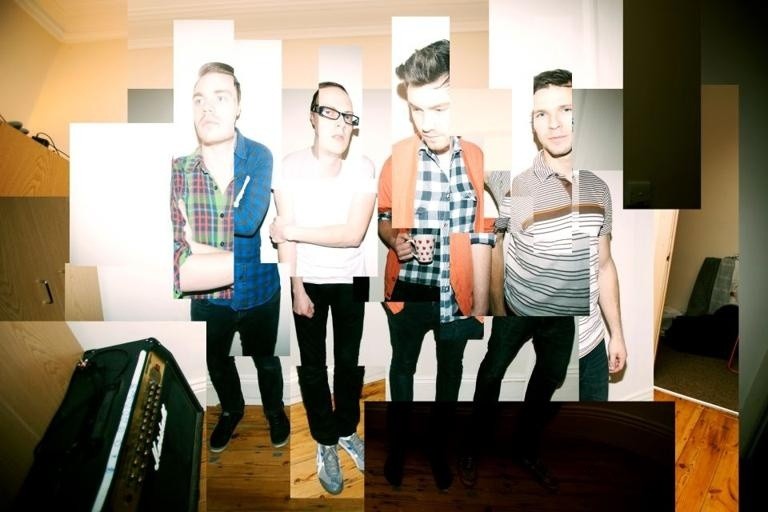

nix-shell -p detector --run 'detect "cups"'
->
[405,234,437,266]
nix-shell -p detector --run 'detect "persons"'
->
[376,36,492,490]
[455,67,629,491]
[269,77,383,499]
[167,62,298,452]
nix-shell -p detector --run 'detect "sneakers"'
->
[315,443,343,495]
[337,432,364,472]
[209,408,245,454]
[263,400,290,448]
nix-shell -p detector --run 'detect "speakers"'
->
[9,337,204,512]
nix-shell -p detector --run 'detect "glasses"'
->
[310,104,360,127]
